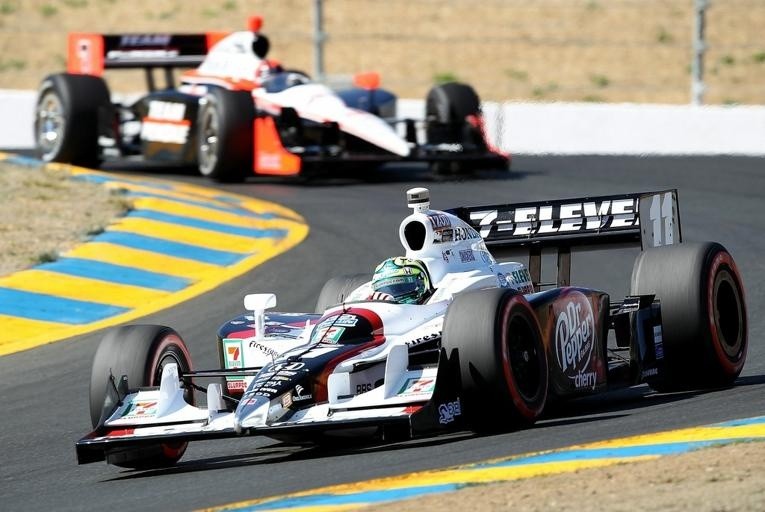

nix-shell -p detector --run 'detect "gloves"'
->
[367,292,394,302]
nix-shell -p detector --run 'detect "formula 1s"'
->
[28,24,515,189]
[71,182,751,475]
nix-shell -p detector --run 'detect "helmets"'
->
[371,255,429,305]
[255,58,286,80]
